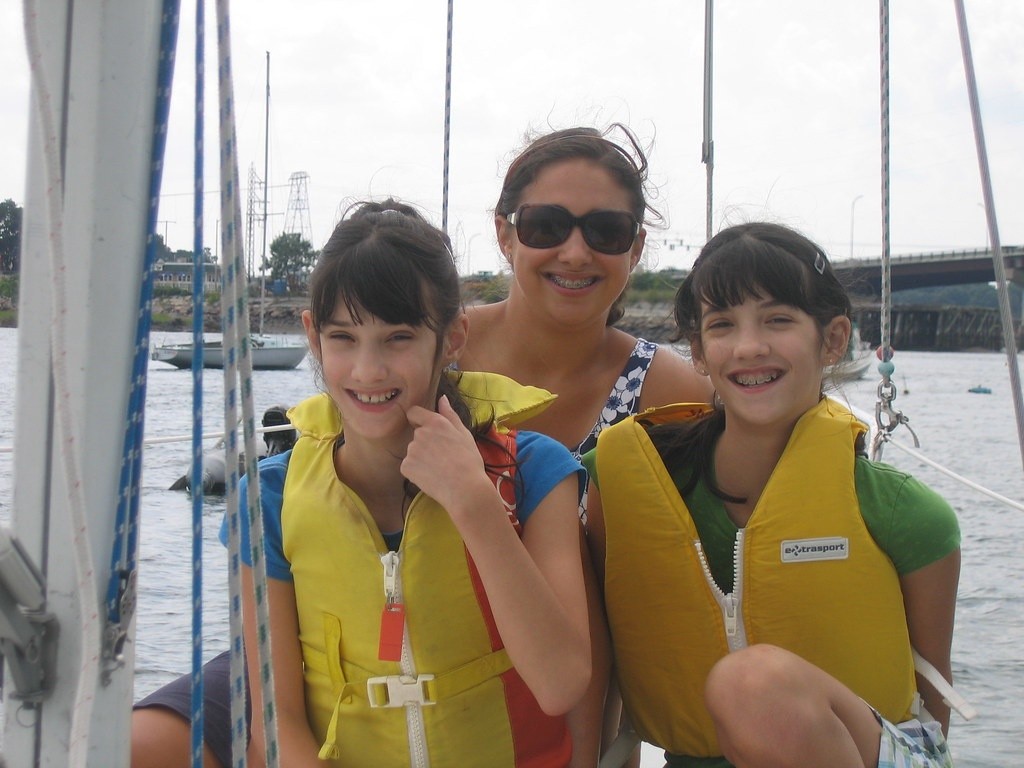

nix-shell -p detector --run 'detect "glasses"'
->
[507,202,640,254]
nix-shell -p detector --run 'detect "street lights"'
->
[849,194,866,260]
[467,232,480,276]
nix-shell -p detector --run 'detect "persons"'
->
[130,126,708,767]
[587,224,959,768]
[220,199,584,768]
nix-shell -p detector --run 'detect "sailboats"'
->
[145,49,310,373]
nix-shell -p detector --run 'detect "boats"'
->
[185,401,300,493]
[821,361,873,383]
